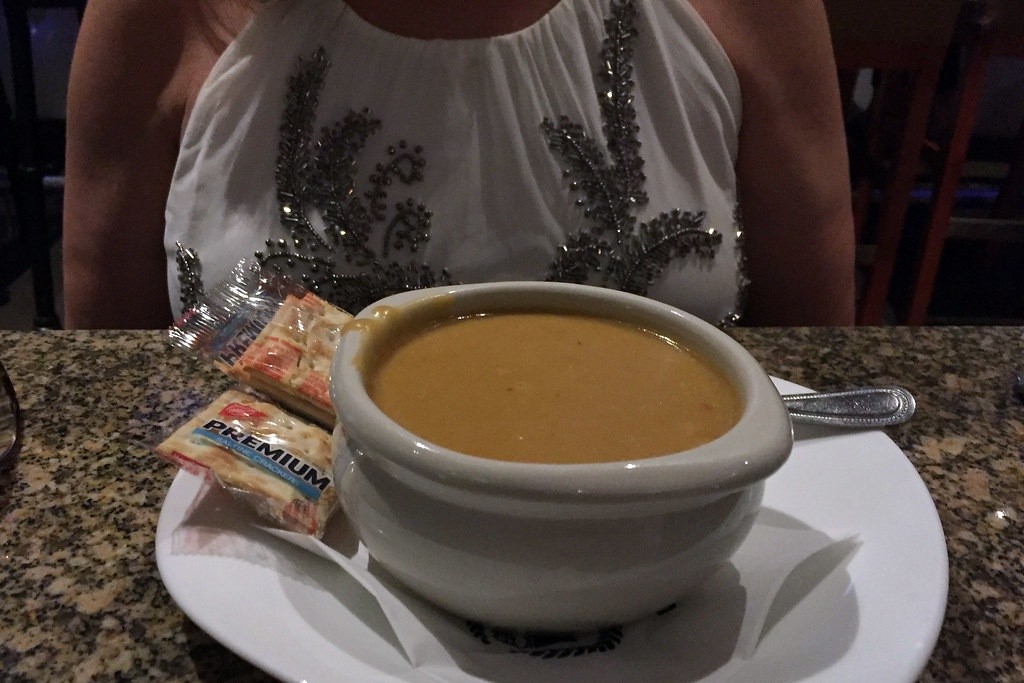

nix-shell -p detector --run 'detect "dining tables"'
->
[0,315,1023,683]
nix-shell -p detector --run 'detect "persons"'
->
[61,0,857,326]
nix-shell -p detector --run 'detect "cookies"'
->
[231,292,356,428]
[154,382,342,541]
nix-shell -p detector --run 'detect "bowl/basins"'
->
[333,278,794,633]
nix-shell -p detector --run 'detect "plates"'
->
[155,373,949,683]
